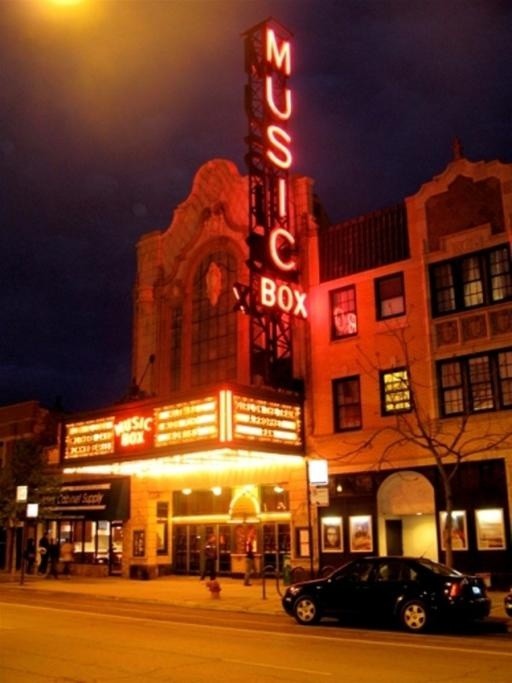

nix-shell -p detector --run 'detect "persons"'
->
[38,531,51,575]
[243,532,259,586]
[23,536,37,575]
[60,538,76,577]
[200,538,217,580]
[324,526,339,549]
[43,538,62,581]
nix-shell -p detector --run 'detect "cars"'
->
[502,589,512,616]
[280,550,496,636]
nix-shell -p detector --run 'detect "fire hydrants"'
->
[207,580,222,599]
